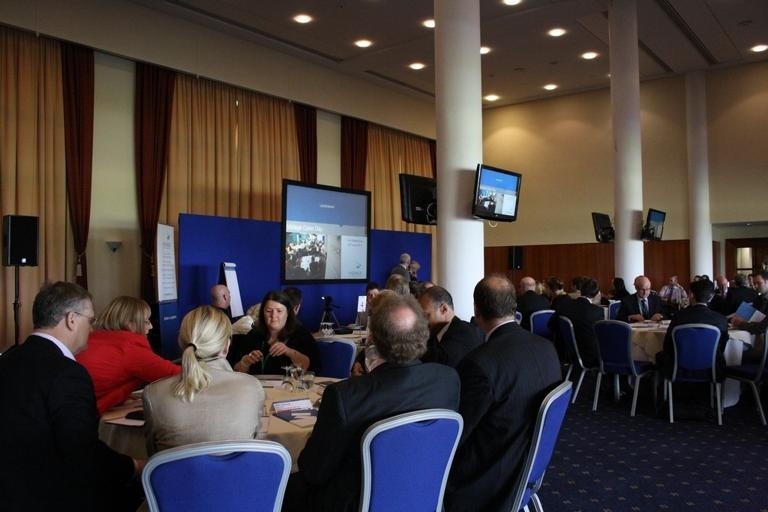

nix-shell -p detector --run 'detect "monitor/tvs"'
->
[473,165,522,222]
[592,212,615,243]
[642,208,666,240]
[399,173,437,225]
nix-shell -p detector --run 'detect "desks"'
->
[97,371,347,474]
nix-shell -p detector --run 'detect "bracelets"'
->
[239,353,252,369]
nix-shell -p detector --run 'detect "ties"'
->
[640,299,649,320]
[668,287,675,304]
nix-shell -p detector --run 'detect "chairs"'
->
[142,438,292,512]
[530,309,556,339]
[551,314,620,406]
[662,323,724,426]
[515,311,522,325]
[592,319,659,417]
[309,335,356,380]
[716,337,768,426]
[359,406,466,512]
[499,380,572,510]
[609,300,622,320]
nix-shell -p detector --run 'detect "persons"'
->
[285,232,327,280]
[229,290,319,378]
[298,289,462,512]
[230,301,263,334]
[209,284,256,323]
[73,294,182,418]
[283,286,305,319]
[138,304,266,455]
[442,270,564,512]
[1,277,148,512]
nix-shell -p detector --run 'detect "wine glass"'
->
[282,359,315,393]
[320,321,335,338]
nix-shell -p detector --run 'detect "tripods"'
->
[319,302,341,330]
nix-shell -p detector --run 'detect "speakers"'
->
[2,215,39,266]
[508,246,523,270]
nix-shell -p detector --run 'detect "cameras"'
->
[321,296,333,302]
